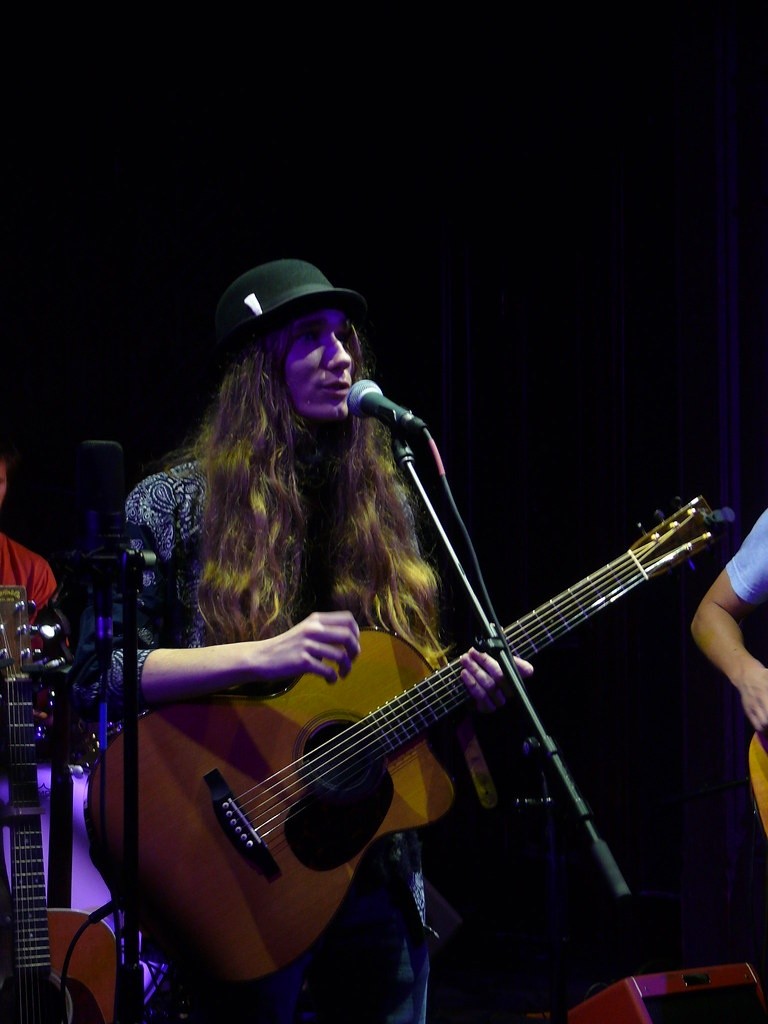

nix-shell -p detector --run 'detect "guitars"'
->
[0,586,122,1021]
[83,485,723,985]
[746,727,768,834]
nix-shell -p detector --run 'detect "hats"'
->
[213,258,369,368]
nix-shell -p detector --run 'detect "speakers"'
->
[569,963,768,1024]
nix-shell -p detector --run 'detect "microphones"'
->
[347,379,428,432]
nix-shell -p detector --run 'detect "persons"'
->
[67,259,535,1024]
[0,441,60,718]
[690,503,768,739]
